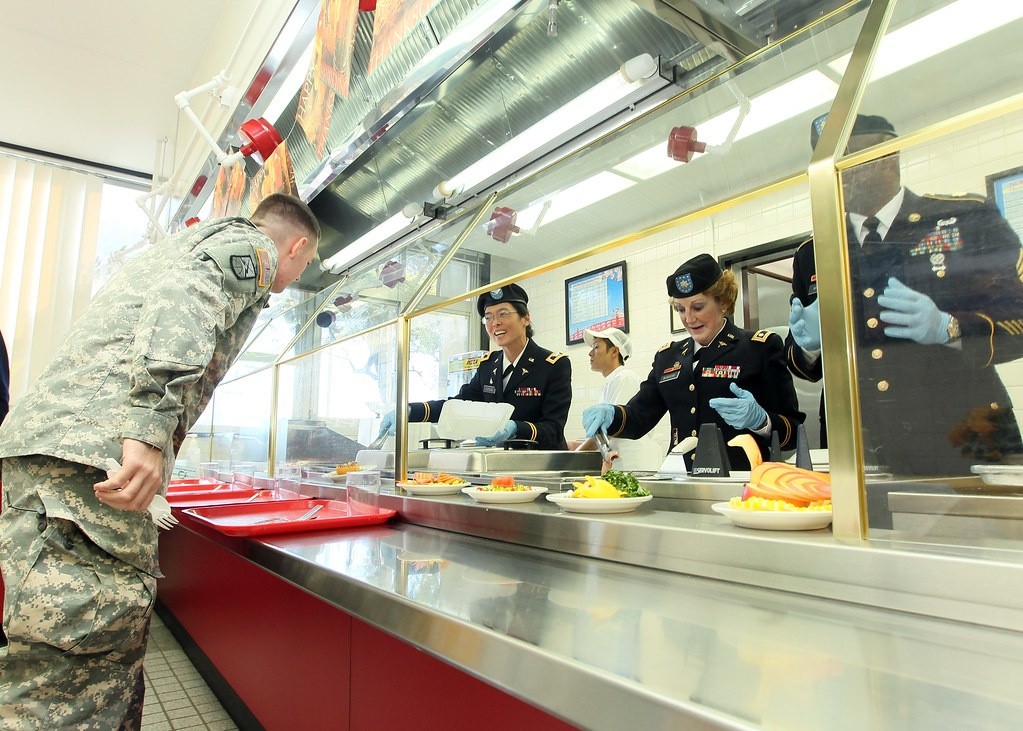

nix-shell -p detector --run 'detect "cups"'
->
[276,467,301,500]
[345,471,381,517]
[233,465,255,490]
[200,462,220,484]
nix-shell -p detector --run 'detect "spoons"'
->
[310,516,320,520]
[107,458,171,519]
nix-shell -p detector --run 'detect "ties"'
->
[861,216,883,263]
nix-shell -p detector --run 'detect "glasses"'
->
[482,310,518,326]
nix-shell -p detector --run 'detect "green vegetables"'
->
[601,471,651,497]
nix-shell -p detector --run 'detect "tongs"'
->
[595,427,619,464]
[368,425,392,451]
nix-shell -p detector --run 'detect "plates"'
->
[711,501,831,531]
[462,486,548,503]
[547,492,653,514]
[396,481,470,495]
[322,465,379,482]
[728,447,829,476]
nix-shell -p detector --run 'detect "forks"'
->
[254,504,323,524]
[213,485,222,491]
[108,470,178,531]
[245,493,260,502]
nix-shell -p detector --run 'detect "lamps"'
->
[431,53,678,207]
[319,202,434,274]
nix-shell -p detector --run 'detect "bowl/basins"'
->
[430,400,515,442]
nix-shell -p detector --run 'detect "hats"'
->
[666,253,723,298]
[583,328,633,368]
[810,114,898,152]
[477,283,529,318]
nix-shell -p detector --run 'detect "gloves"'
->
[708,384,767,431]
[874,274,952,347]
[379,408,411,437]
[788,297,822,353]
[475,420,518,447]
[581,401,615,439]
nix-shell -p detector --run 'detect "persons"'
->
[0,192,321,731]
[582,254,806,470]
[566,327,661,471]
[785,112,1023,476]
[380,283,572,451]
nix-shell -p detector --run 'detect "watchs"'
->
[946,315,961,343]
